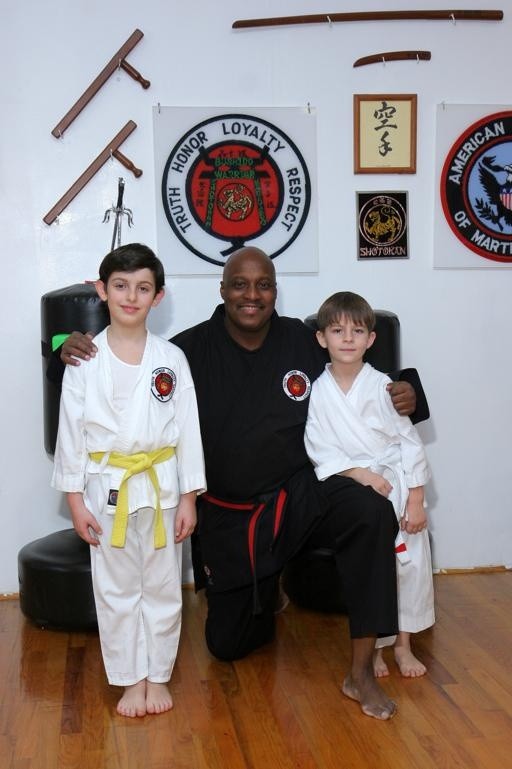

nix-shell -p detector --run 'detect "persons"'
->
[44,246,428,723]
[51,241,212,719]
[304,289,433,680]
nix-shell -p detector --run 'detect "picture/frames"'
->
[356,190,410,262]
[352,93,418,176]
